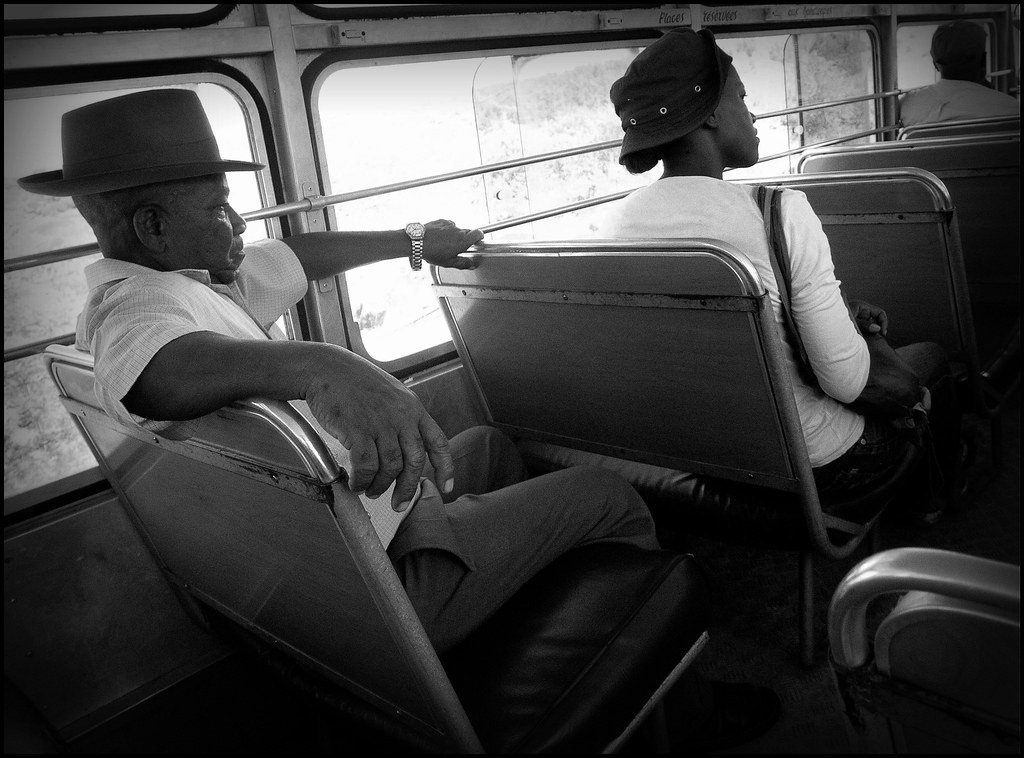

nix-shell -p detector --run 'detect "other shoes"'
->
[669,680,781,756]
[908,472,968,528]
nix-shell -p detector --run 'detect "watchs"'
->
[404,222,426,271]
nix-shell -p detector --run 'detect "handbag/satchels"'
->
[757,185,921,416]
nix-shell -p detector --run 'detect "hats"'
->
[931,20,988,65]
[610,27,733,165]
[16,89,266,197]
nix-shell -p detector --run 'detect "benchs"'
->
[896,114,1021,140]
[723,166,1021,469]
[826,547,1020,755]
[797,130,1021,308]
[430,236,925,671]
[44,343,737,754]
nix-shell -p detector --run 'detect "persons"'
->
[612,27,985,525]
[17,90,781,755]
[897,20,1020,126]
